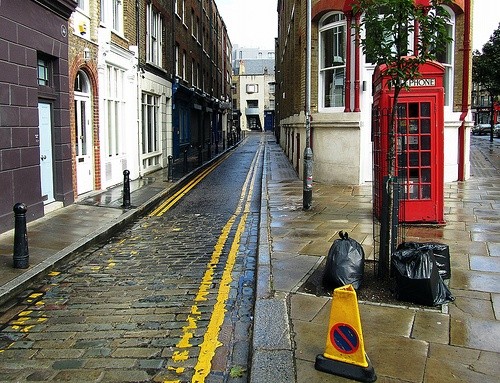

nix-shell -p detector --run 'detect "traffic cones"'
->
[313,283,378,383]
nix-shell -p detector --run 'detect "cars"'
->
[494,123,500,137]
[472,123,493,136]
